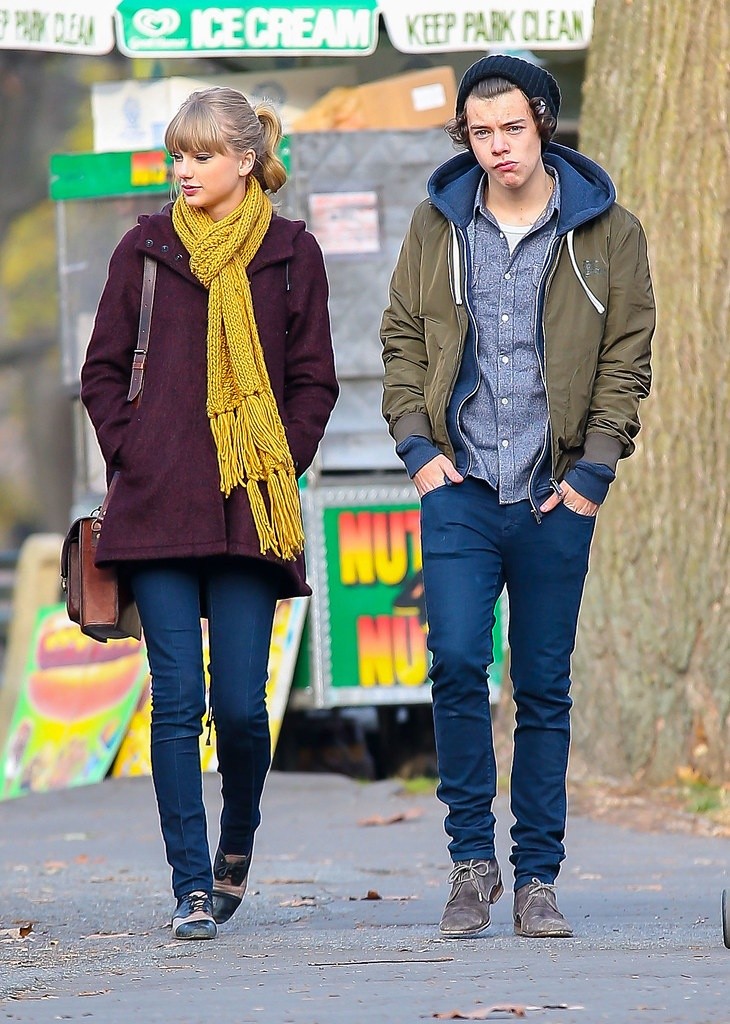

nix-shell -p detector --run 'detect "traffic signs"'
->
[384,0,595,52]
[1,8,116,55]
[116,2,380,57]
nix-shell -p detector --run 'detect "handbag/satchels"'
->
[60,516,142,643]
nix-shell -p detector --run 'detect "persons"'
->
[378,54,658,936]
[79,89,341,938]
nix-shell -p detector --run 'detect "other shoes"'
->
[172,891,217,939]
[513,878,574,937]
[439,856,504,934]
[211,846,252,924]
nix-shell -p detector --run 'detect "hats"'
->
[456,55,562,155]
